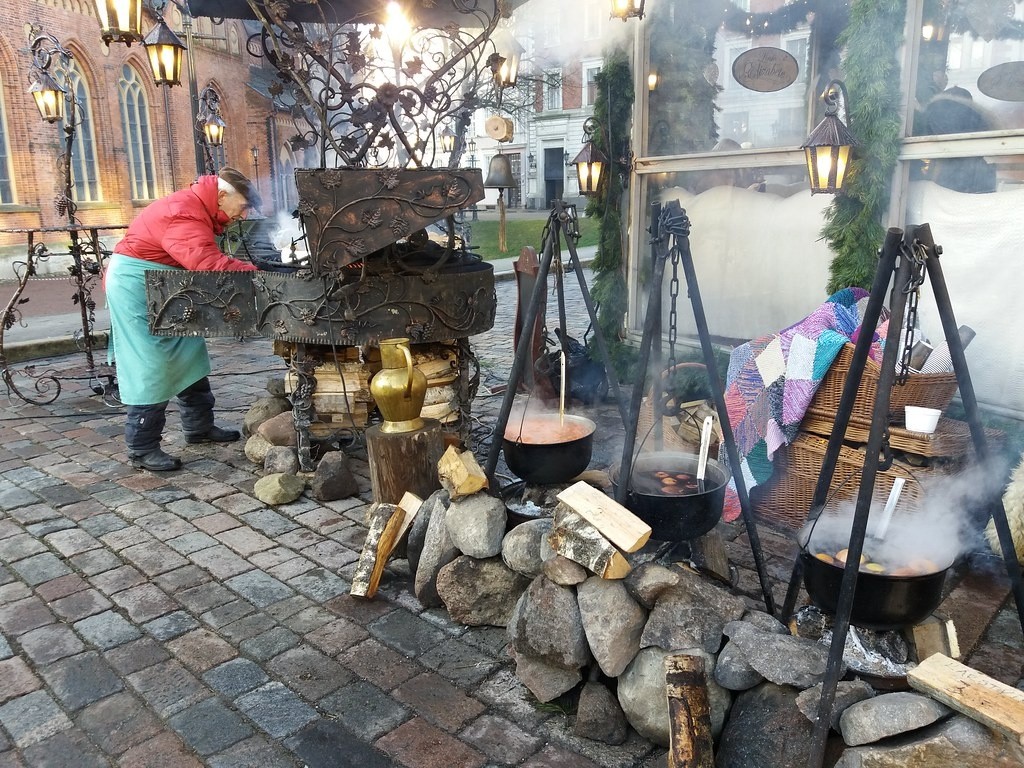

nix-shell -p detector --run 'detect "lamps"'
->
[570,119,611,195]
[799,77,867,192]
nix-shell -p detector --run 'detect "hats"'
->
[219,166,263,216]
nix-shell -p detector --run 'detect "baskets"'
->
[748,343,1007,543]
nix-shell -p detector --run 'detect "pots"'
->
[793,462,971,630]
[607,404,731,541]
[502,372,596,485]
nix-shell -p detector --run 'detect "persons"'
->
[100,165,264,472]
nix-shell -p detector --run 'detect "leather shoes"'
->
[128,426,241,471]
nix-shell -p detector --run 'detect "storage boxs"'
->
[711,408,1010,547]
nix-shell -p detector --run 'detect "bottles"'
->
[550,353,605,404]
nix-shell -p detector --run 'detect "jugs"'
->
[371,338,427,434]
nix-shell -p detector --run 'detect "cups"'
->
[895,323,977,379]
[903,406,942,434]
